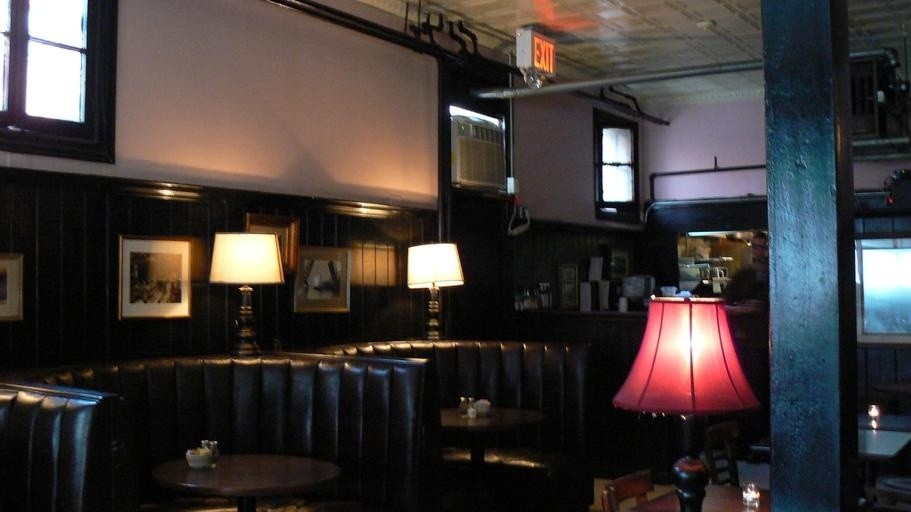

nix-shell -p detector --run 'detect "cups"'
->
[742,481,760,509]
[618,297,628,313]
[865,404,882,430]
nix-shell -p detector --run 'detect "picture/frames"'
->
[117,235,193,321]
[245,212,352,314]
[0,252,25,322]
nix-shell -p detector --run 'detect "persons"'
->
[719,228,768,332]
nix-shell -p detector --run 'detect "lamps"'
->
[208,233,286,357]
[611,296,759,511]
[407,242,466,340]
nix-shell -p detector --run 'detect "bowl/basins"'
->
[185,451,210,468]
[474,398,491,416]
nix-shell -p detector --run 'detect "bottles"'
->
[457,396,477,418]
[513,282,553,311]
[201,439,220,468]
